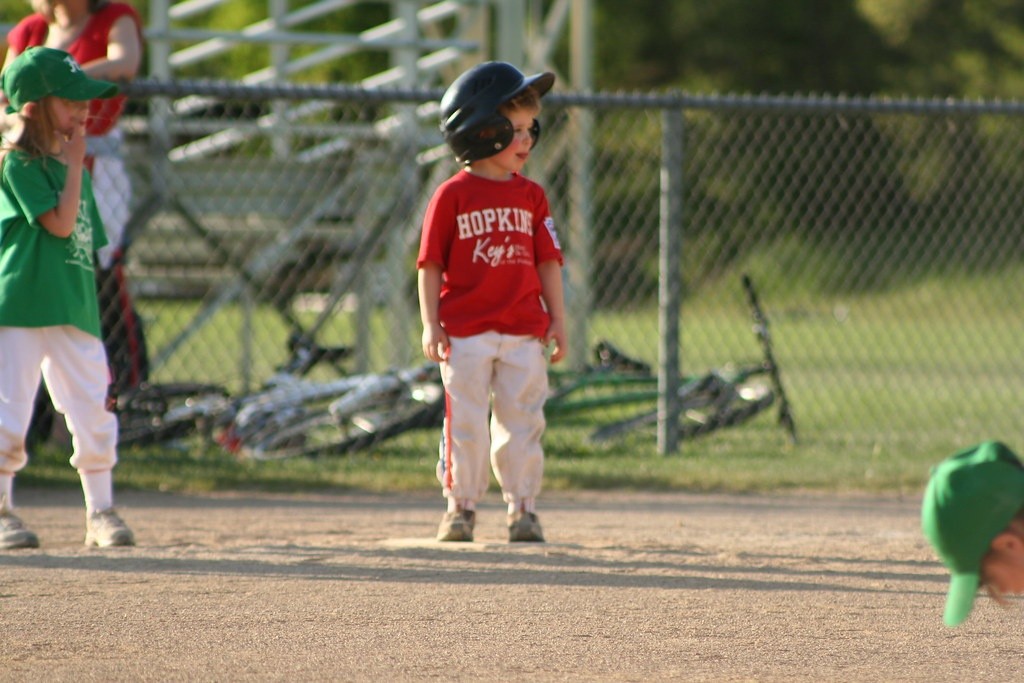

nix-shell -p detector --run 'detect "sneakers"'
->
[507,508,546,542]
[0,513,38,549]
[85,511,135,547]
[438,510,475,542]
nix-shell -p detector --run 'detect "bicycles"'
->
[39,264,806,481]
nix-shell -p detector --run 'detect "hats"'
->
[4,46,121,113]
[921,440,1023,627]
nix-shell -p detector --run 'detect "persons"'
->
[1,45,137,550]
[917,439,1024,626]
[0,1,153,445]
[412,61,567,542]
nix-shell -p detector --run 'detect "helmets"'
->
[438,62,555,164]
